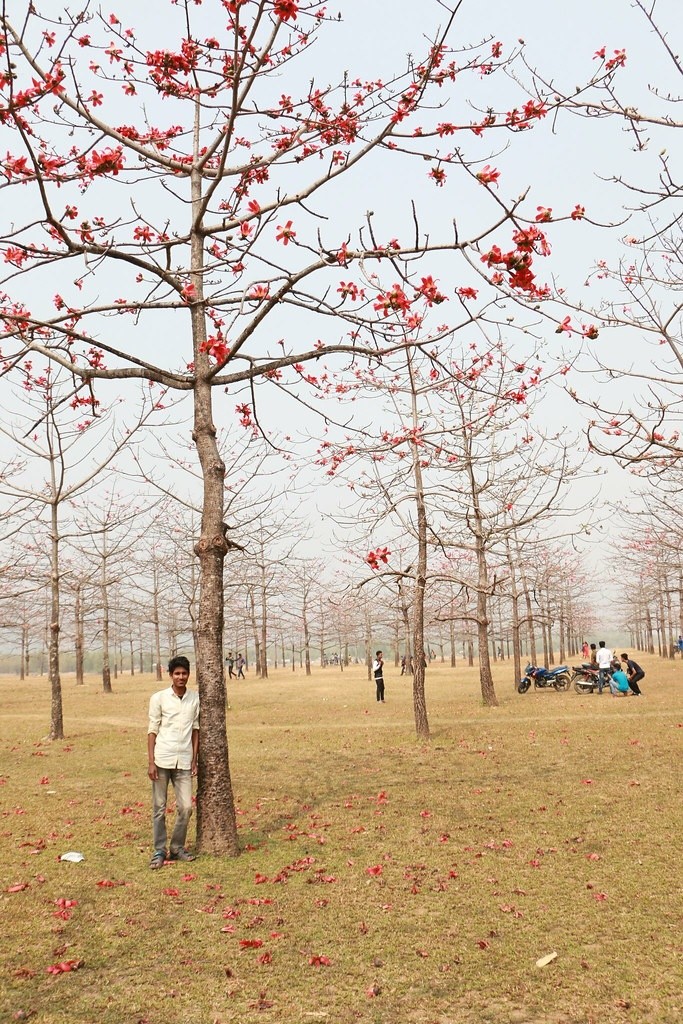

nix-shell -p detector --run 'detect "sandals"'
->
[149,853,166,870]
[169,850,196,861]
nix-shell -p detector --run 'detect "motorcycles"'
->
[517,660,572,694]
[569,650,624,694]
[400,655,413,676]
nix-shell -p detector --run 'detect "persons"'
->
[224,652,246,681]
[372,651,386,704]
[498,647,501,658]
[148,655,201,870]
[673,636,683,659]
[323,650,437,677]
[581,642,645,697]
